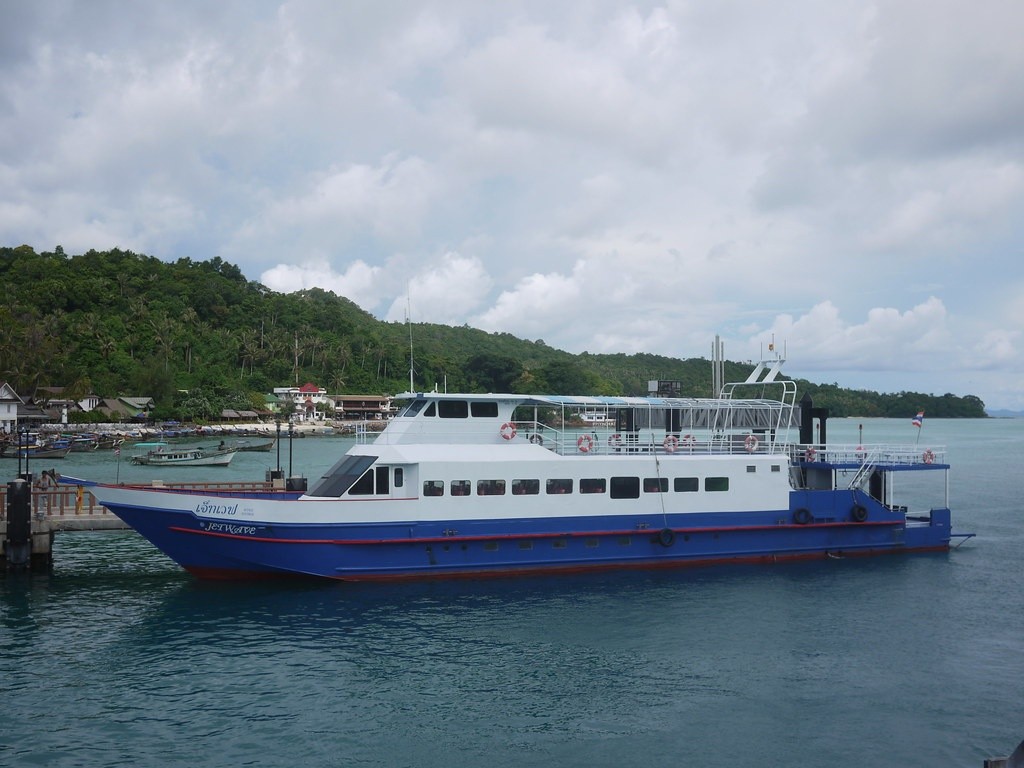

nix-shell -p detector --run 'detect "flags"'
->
[912,411,926,428]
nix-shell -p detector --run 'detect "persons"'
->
[33,469,59,515]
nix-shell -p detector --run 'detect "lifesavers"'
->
[500,423,935,465]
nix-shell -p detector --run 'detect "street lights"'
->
[287,421,296,478]
[274,420,282,479]
[24,423,31,484]
[17,426,23,479]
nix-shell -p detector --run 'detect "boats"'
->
[0,440,72,458]
[236,440,275,452]
[99,420,304,449]
[40,433,98,452]
[129,441,240,467]
[53,331,978,590]
